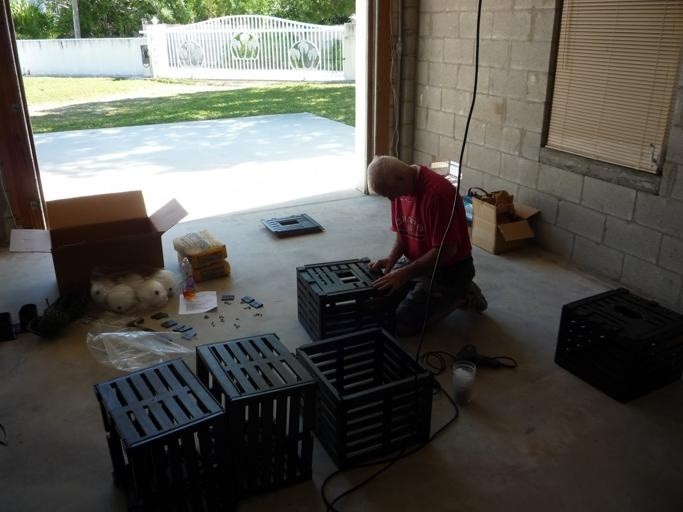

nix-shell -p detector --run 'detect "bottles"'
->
[179,256,194,300]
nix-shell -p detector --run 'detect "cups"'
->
[451,360,476,405]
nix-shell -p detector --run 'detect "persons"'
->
[363,153,488,338]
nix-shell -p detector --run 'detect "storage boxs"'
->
[9,189,190,298]
[469,195,547,257]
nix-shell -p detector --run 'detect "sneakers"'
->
[464,280,489,314]
